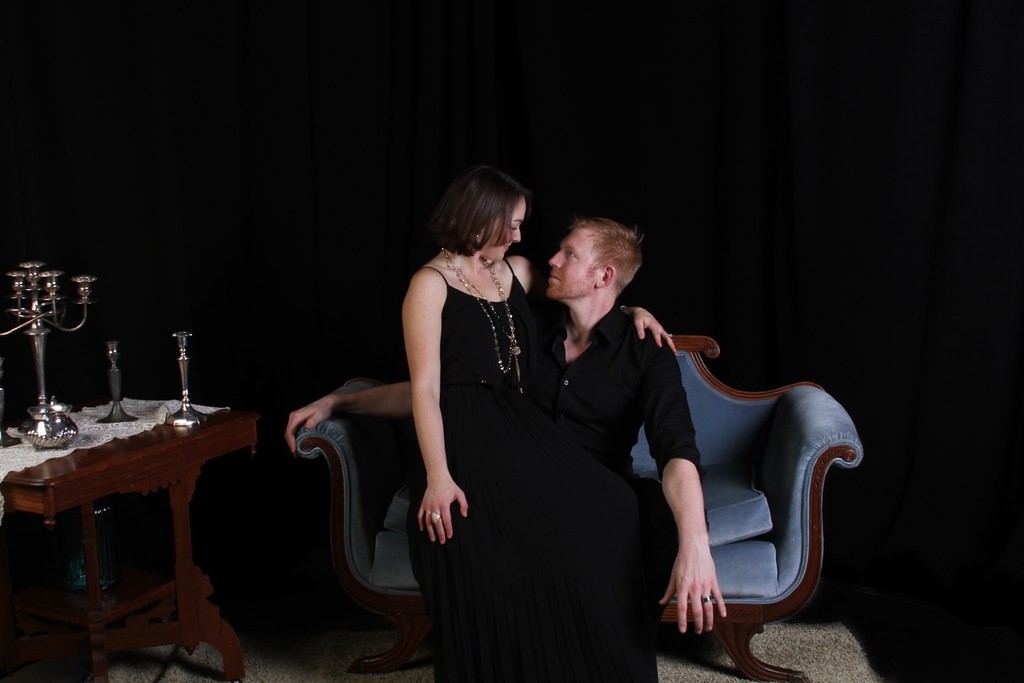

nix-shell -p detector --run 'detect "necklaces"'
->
[442,248,522,392]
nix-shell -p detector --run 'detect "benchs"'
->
[295,335,864,683]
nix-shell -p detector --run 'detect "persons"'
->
[400,166,661,683]
[284,215,728,633]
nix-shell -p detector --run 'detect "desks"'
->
[0,398,258,683]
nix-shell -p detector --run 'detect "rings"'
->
[669,334,672,337]
[705,596,713,603]
[432,512,442,523]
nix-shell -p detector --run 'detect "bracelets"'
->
[620,305,627,310]
